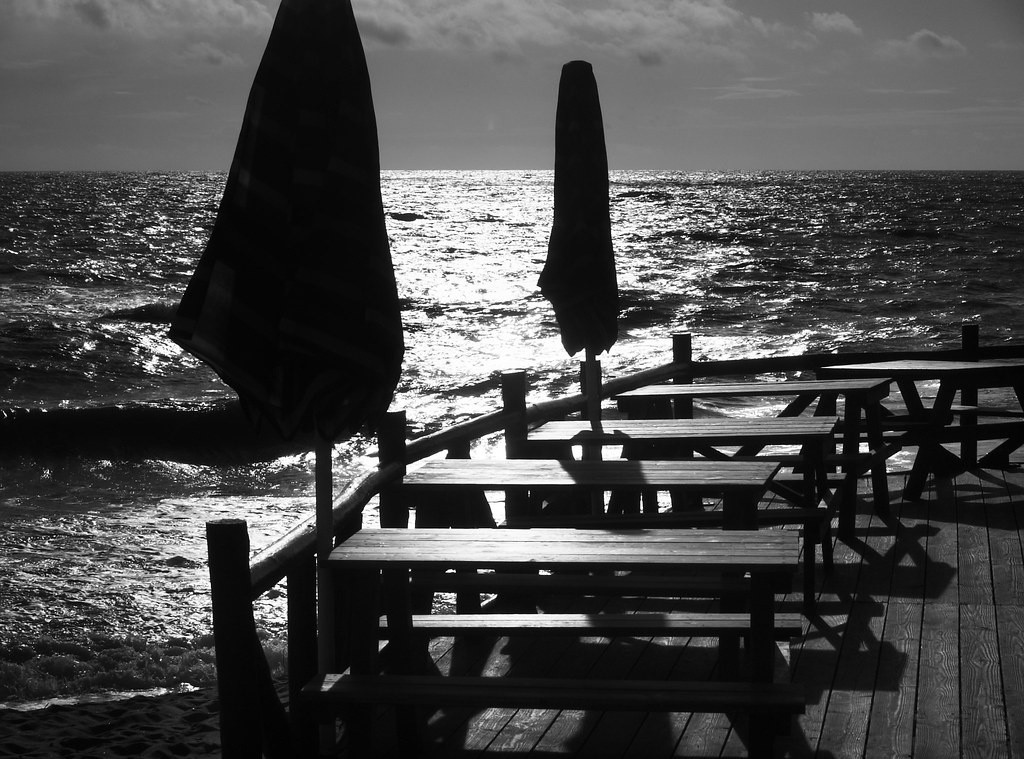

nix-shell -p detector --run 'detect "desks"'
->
[525,416,846,577]
[331,525,805,757]
[391,456,784,615]
[798,360,1024,500]
[612,378,894,534]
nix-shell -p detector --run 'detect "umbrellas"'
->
[167,0,406,758]
[538,60,620,427]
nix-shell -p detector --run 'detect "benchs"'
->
[416,403,1024,602]
[376,611,805,637]
[298,672,805,714]
